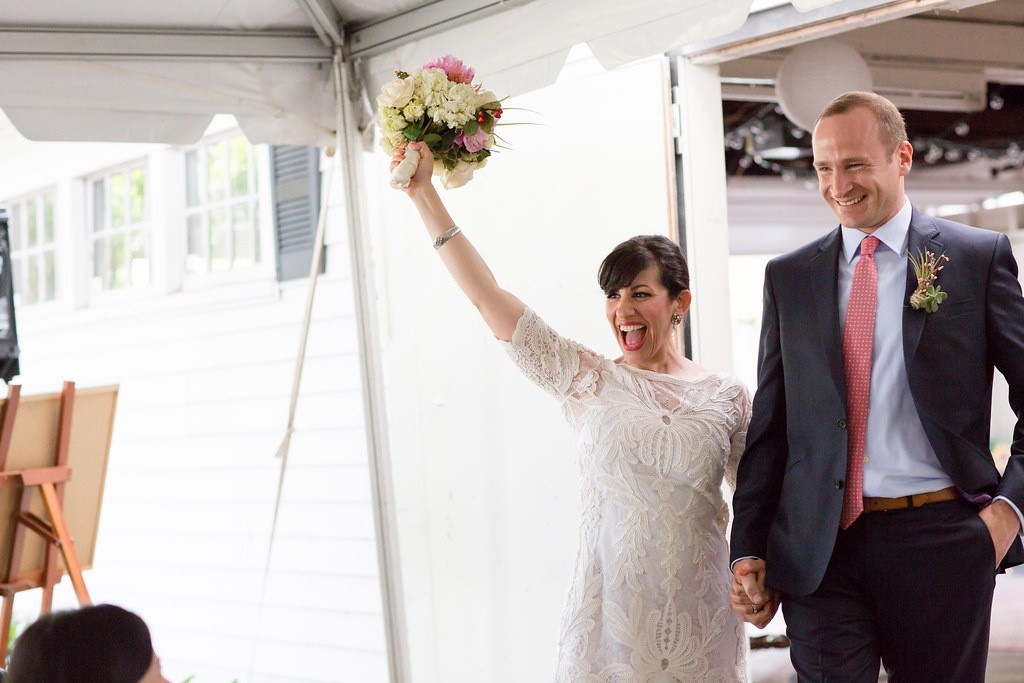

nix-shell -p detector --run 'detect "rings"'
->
[751,604,757,614]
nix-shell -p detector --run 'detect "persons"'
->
[391,141,773,683]
[730,92,1023,683]
[5,605,169,683]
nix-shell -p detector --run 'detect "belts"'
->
[862,488,959,511]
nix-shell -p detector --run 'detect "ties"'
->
[840,234,881,529]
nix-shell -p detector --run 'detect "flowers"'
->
[906,246,949,313]
[374,54,542,190]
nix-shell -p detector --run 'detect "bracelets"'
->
[433,226,459,251]
